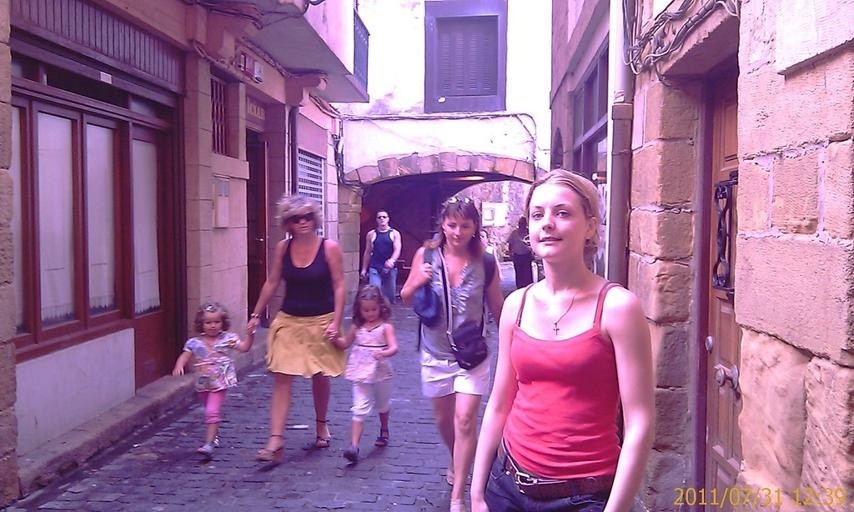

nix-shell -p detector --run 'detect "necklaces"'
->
[551,284,585,335]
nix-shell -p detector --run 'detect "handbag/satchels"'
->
[412,244,439,326]
[438,243,488,370]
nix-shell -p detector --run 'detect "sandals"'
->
[256,434,285,465]
[197,442,216,458]
[374,426,389,447]
[450,493,466,512]
[343,445,359,464]
[214,434,221,447]
[314,417,331,449]
[447,454,456,485]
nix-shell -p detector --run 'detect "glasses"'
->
[375,216,388,219]
[285,211,314,223]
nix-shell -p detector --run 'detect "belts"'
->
[497,440,616,500]
[368,265,397,270]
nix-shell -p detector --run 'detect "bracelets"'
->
[250,313,262,319]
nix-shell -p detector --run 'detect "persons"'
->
[249,195,346,466]
[402,195,505,512]
[171,301,256,456]
[360,209,402,306]
[324,284,400,463]
[507,216,536,289]
[470,168,658,512]
[479,229,503,280]
